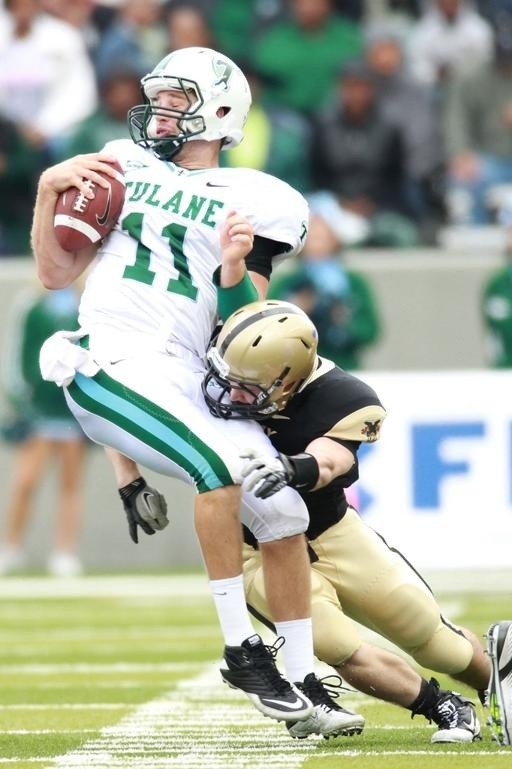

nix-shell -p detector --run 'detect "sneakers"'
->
[488,619,512,744]
[218,633,315,721]
[412,677,482,743]
[285,672,366,738]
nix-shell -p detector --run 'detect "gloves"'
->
[118,477,169,544]
[239,446,318,499]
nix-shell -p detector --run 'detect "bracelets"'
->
[288,450,321,495]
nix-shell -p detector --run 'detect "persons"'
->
[2,273,92,582]
[480,618,512,752]
[101,296,493,752]
[29,44,365,740]
[0,1,511,368]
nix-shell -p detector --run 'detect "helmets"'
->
[202,299,320,421]
[138,45,253,150]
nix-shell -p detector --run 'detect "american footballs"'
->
[54,159,125,252]
